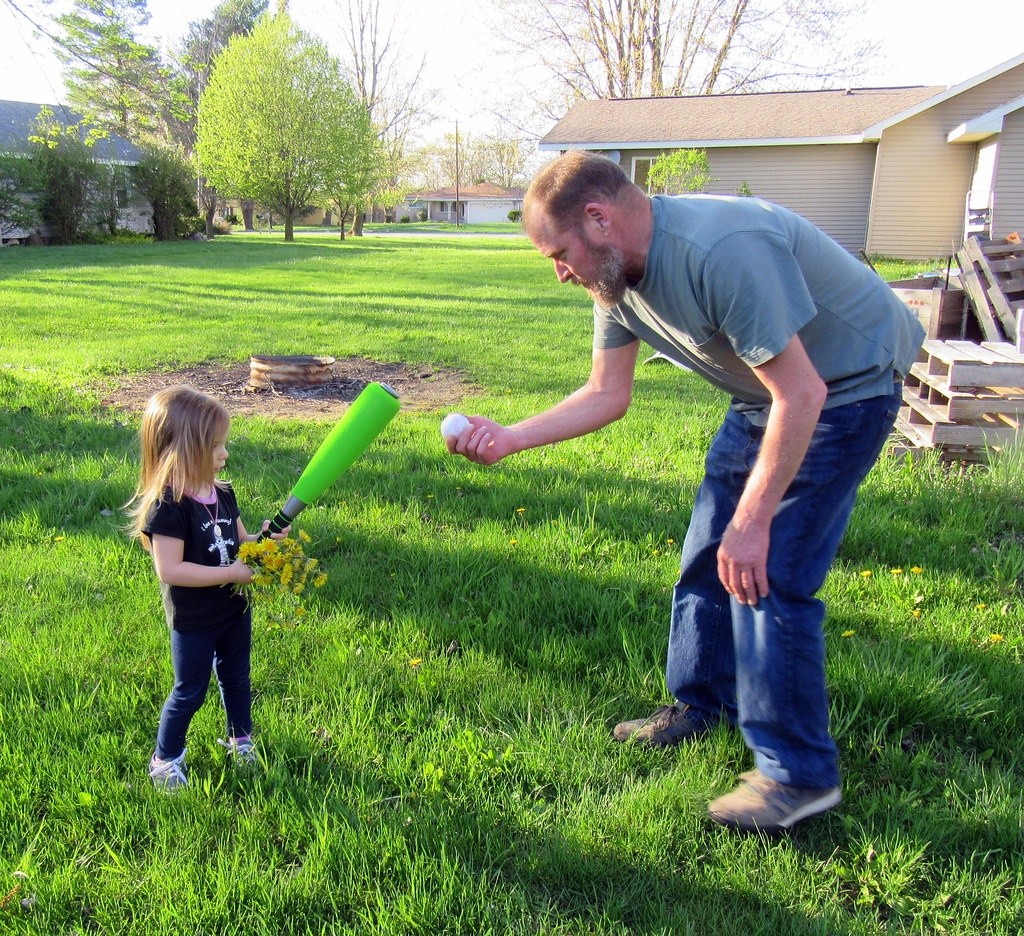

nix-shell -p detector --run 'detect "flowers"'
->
[220,528,327,631]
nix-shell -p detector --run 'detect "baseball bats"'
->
[242,381,401,569]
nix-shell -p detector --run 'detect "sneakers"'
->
[217,738,257,781]
[708,769,842,831]
[613,705,735,752]
[149,747,188,795]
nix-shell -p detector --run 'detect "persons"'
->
[123,386,293,795]
[445,147,926,833]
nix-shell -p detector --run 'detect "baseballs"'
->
[440,413,469,439]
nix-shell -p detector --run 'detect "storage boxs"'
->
[883,276,973,341]
